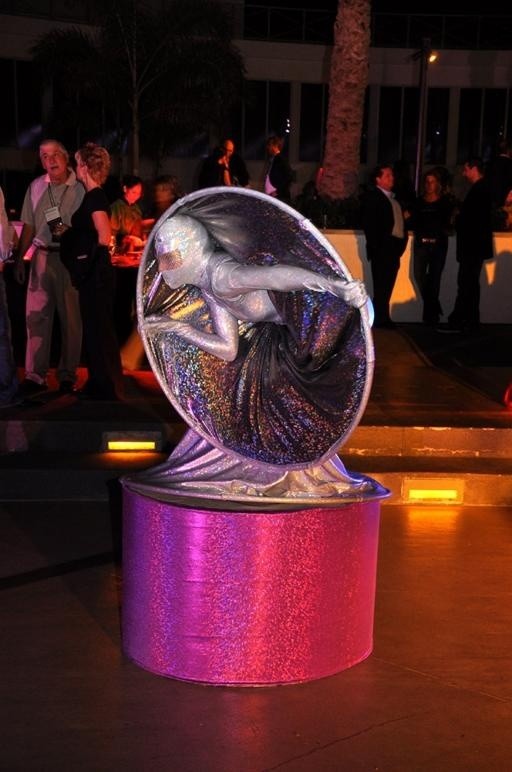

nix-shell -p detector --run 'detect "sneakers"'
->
[20,376,128,402]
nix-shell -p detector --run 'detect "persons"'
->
[263,135,294,205]
[408,168,458,327]
[145,174,184,219]
[200,138,250,187]
[488,141,512,227]
[362,163,410,329]
[438,155,499,333]
[12,139,85,395]
[0,184,26,409]
[108,176,146,247]
[118,213,395,500]
[52,144,131,403]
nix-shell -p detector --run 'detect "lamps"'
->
[102,429,164,454]
